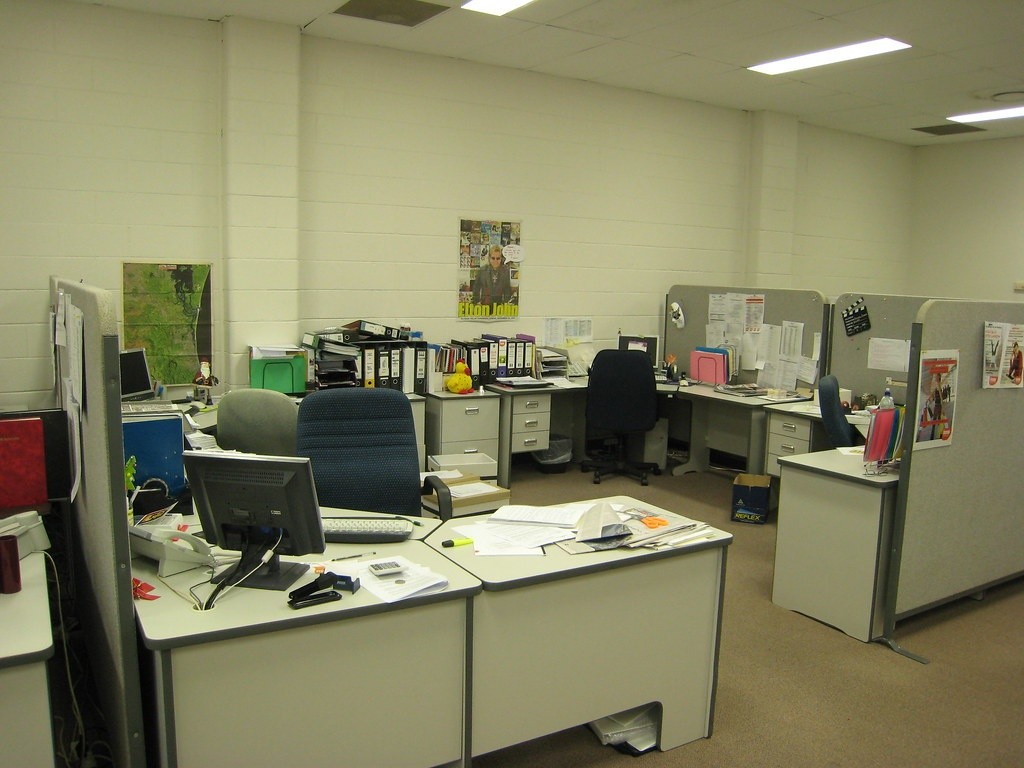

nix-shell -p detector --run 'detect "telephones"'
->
[128,525,213,577]
[0,511,52,560]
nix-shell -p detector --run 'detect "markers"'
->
[442,537,473,548]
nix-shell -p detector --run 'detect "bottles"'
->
[880,391,894,409]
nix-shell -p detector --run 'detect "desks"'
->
[0,282,1024,768]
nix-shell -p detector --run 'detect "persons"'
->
[926,374,945,440]
[990,340,1000,368]
[459,220,520,315]
[1009,342,1022,385]
[194,357,218,405]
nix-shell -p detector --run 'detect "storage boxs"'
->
[464,338,490,385]
[427,452,498,486]
[481,334,507,377]
[353,350,363,388]
[451,339,481,390]
[341,320,411,341]
[372,343,389,388]
[388,344,402,392]
[507,339,516,377]
[414,345,427,395]
[732,474,770,524]
[302,333,360,358]
[522,339,533,376]
[513,340,524,376]
[361,343,375,388]
[476,339,498,384]
[536,346,571,382]
[427,348,467,391]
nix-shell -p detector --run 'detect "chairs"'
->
[818,375,855,449]
[216,389,297,457]
[296,387,453,521]
[580,348,661,486]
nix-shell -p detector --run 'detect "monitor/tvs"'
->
[181,449,325,592]
[119,347,154,401]
[617,335,660,369]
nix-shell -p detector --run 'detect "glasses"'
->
[491,257,501,261]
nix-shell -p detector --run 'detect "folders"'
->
[691,346,729,384]
[863,409,895,461]
[451,334,532,389]
[354,346,427,395]
[0,408,71,508]
[302,320,400,357]
[122,415,185,489]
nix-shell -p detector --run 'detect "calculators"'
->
[368,562,404,576]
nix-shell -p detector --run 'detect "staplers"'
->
[287,572,342,610]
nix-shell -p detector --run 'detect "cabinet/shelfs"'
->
[512,394,551,452]
[425,391,500,474]
[766,412,830,476]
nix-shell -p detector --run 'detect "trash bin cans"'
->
[539,435,570,474]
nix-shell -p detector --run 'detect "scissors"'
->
[627,513,668,528]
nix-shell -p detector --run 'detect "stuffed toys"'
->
[443,359,474,394]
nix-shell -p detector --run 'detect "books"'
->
[134,502,183,532]
[714,382,768,397]
[494,375,555,391]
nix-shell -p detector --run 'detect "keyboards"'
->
[121,400,174,413]
[655,375,667,384]
[320,520,414,544]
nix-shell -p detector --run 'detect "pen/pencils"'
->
[331,552,376,562]
[153,382,164,396]
[662,361,670,367]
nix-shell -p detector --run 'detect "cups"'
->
[0,536,21,594]
